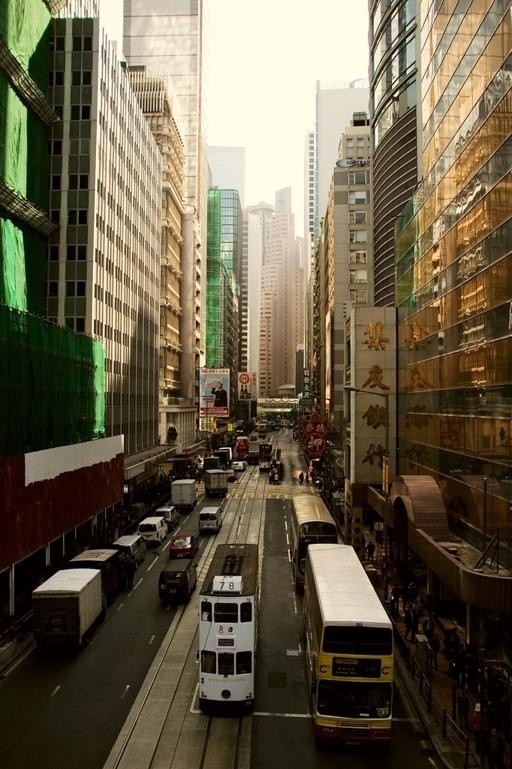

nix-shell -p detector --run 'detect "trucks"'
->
[69,549,127,601]
[31,567,107,647]
[205,469,228,501]
[172,478,198,512]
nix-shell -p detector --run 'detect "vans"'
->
[199,507,225,531]
[114,504,181,570]
[156,556,198,600]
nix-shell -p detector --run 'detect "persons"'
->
[210,382,227,407]
[360,523,511,769]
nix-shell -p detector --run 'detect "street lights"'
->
[344,382,393,607]
[309,393,331,431]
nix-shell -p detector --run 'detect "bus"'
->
[290,496,339,588]
[191,540,264,720]
[302,543,393,750]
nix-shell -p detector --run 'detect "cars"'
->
[204,415,295,486]
[171,534,201,558]
